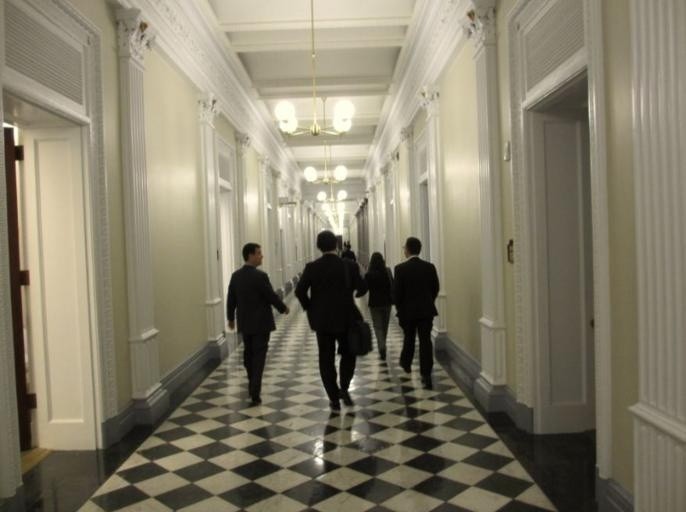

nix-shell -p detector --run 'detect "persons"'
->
[225,242,290,404]
[295,230,365,408]
[393,237,439,376]
[340,244,356,262]
[364,251,394,360]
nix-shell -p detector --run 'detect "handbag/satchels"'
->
[337,322,373,356]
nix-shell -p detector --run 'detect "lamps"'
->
[275,1,356,138]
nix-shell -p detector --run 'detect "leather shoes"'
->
[252,396,262,403]
[339,389,353,406]
[330,402,340,409]
[400,360,411,373]
